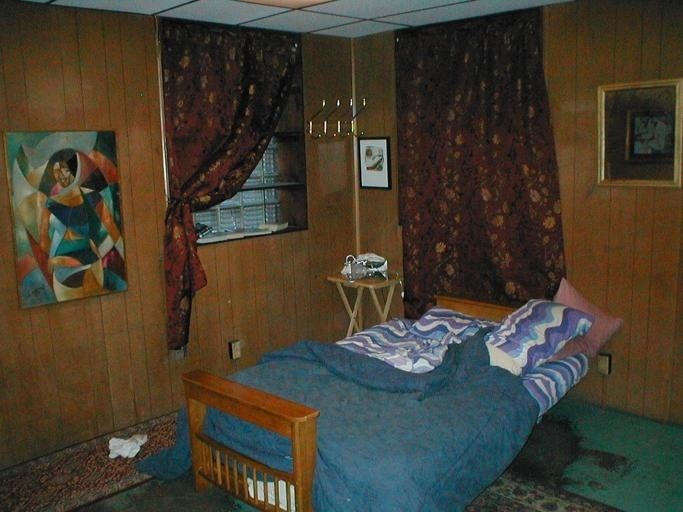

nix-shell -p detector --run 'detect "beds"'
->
[181,293,589,512]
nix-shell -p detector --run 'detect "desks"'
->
[326,273,400,338]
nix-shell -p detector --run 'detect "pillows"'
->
[409,276,622,379]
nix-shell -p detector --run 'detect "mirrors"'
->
[597,78,681,188]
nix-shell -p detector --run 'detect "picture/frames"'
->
[2,129,129,309]
[624,108,674,163]
[357,137,392,190]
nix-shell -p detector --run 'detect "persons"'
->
[365,145,384,171]
[38,147,127,302]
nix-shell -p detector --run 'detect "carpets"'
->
[0,409,625,512]
